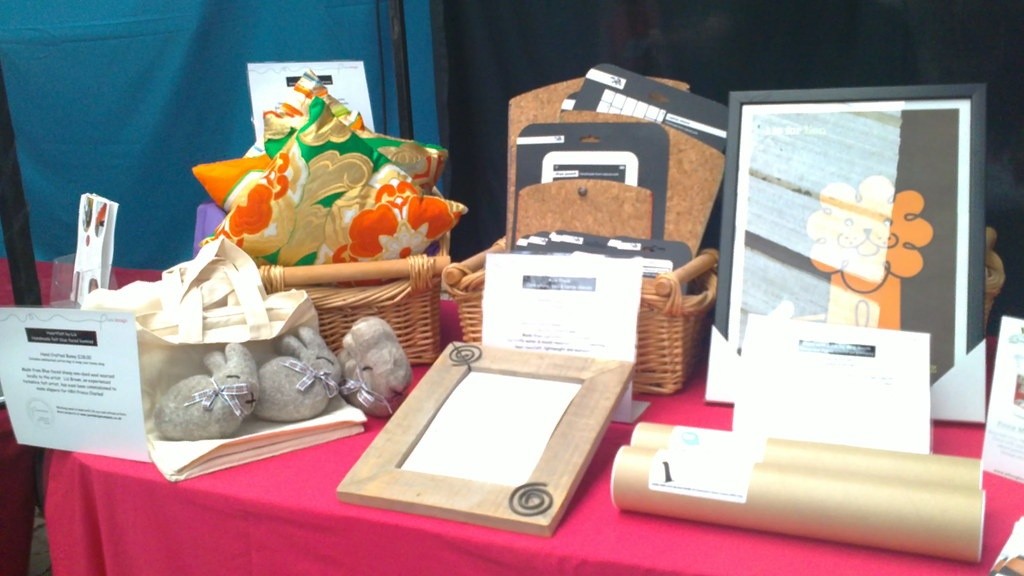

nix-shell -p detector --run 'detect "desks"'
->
[0,254,1024,576]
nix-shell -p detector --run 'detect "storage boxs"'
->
[257,229,456,370]
[443,235,724,403]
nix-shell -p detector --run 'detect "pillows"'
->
[190,68,451,217]
[198,97,469,267]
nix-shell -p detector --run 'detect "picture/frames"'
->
[701,80,984,427]
[333,338,636,542]
